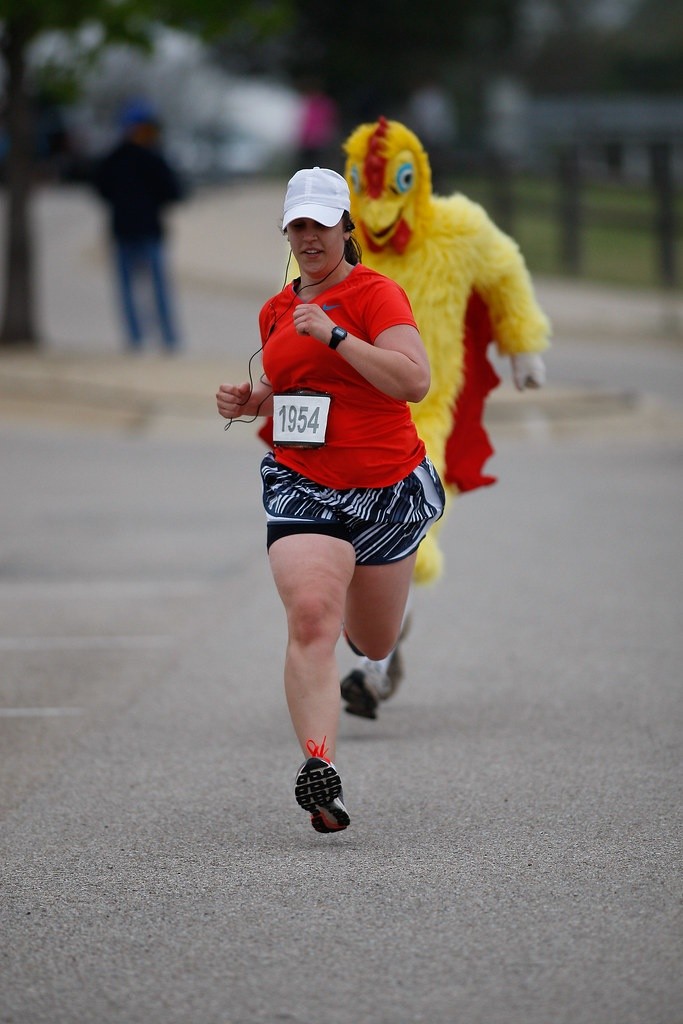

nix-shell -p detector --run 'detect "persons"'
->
[215,165,446,833]
[284,116,549,721]
[94,100,186,350]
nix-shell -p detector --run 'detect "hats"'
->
[122,105,156,127]
[282,167,350,230]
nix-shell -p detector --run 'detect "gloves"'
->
[512,354,549,394]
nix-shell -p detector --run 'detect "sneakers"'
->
[382,611,411,703]
[295,735,350,834]
[342,668,378,719]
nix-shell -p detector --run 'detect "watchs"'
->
[329,326,347,349]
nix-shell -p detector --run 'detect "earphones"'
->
[345,223,356,233]
